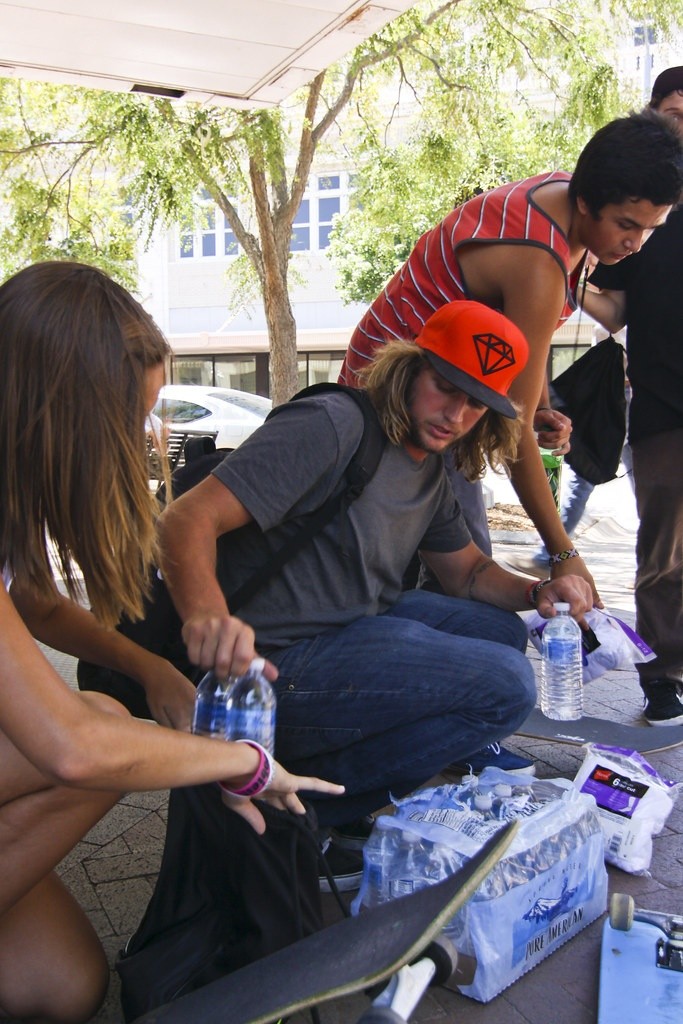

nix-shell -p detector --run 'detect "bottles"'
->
[540,602,584,720]
[362,775,608,1002]
[538,425,562,517]
[193,658,276,760]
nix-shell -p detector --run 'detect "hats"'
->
[417,300,531,420]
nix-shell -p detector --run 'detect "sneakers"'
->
[313,838,365,893]
[643,680,683,727]
[330,812,378,852]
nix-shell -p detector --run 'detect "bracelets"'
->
[549,549,580,564]
[526,577,552,610]
[217,738,275,798]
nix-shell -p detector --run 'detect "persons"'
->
[77,303,595,897]
[0,259,346,1024]
[335,104,683,580]
[573,66,683,726]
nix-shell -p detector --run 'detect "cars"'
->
[144,385,273,467]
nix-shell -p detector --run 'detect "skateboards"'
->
[129,818,521,1024]
[597,892,683,1024]
[512,707,683,755]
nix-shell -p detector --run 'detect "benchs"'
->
[145,428,218,480]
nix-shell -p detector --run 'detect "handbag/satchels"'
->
[548,335,628,483]
[77,382,388,722]
[114,782,350,1024]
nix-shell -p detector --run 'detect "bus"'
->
[165,319,610,400]
